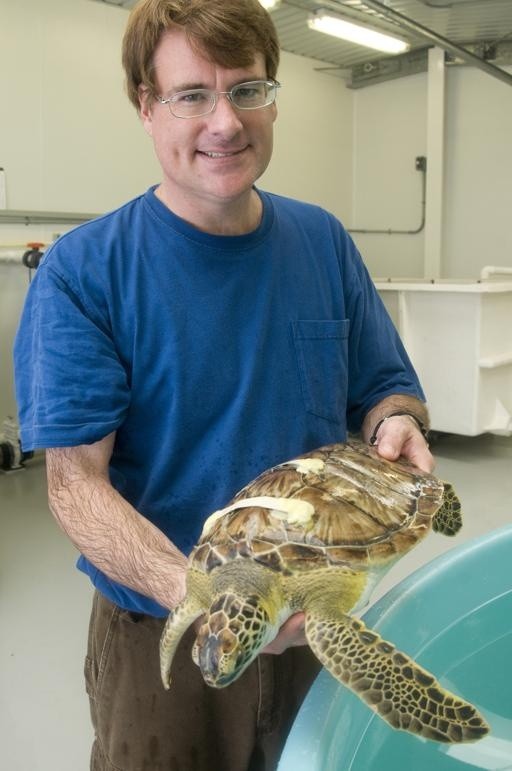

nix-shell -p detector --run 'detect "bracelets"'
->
[370,411,431,452]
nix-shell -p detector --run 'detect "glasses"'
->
[154,75,281,119]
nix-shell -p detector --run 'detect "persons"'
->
[14,1,436,771]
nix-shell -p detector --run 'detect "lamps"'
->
[261,3,277,16]
[307,10,409,61]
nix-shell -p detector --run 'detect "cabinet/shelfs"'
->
[376,282,510,435]
